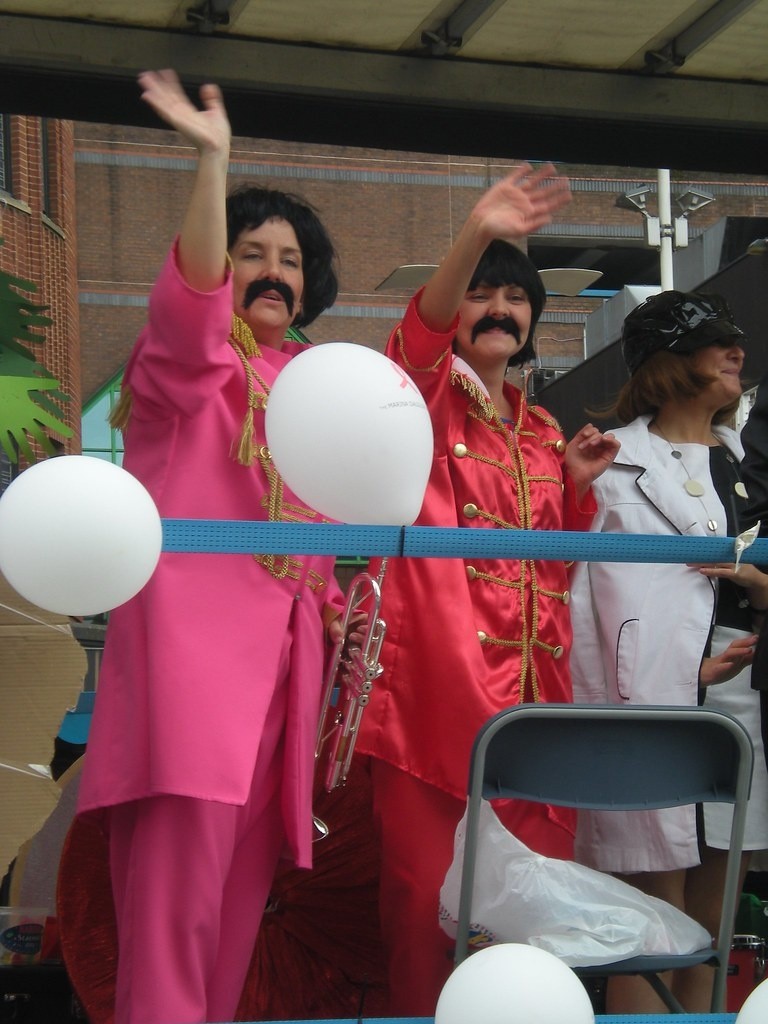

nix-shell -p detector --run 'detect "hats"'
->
[621,290,749,377]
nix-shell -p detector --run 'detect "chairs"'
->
[449,701,755,1011]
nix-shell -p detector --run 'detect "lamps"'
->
[675,184,719,251]
[612,182,660,252]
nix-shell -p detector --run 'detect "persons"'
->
[77,70,373,1023]
[570,291,768,1014]
[344,162,622,1017]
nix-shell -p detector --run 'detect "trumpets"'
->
[312,555,390,843]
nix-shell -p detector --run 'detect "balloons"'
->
[265,342,434,526]
[435,943,595,1024]
[735,978,768,1024]
[0,456,163,617]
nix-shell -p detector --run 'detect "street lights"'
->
[613,168,718,293]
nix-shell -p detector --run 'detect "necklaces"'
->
[656,421,749,609]
